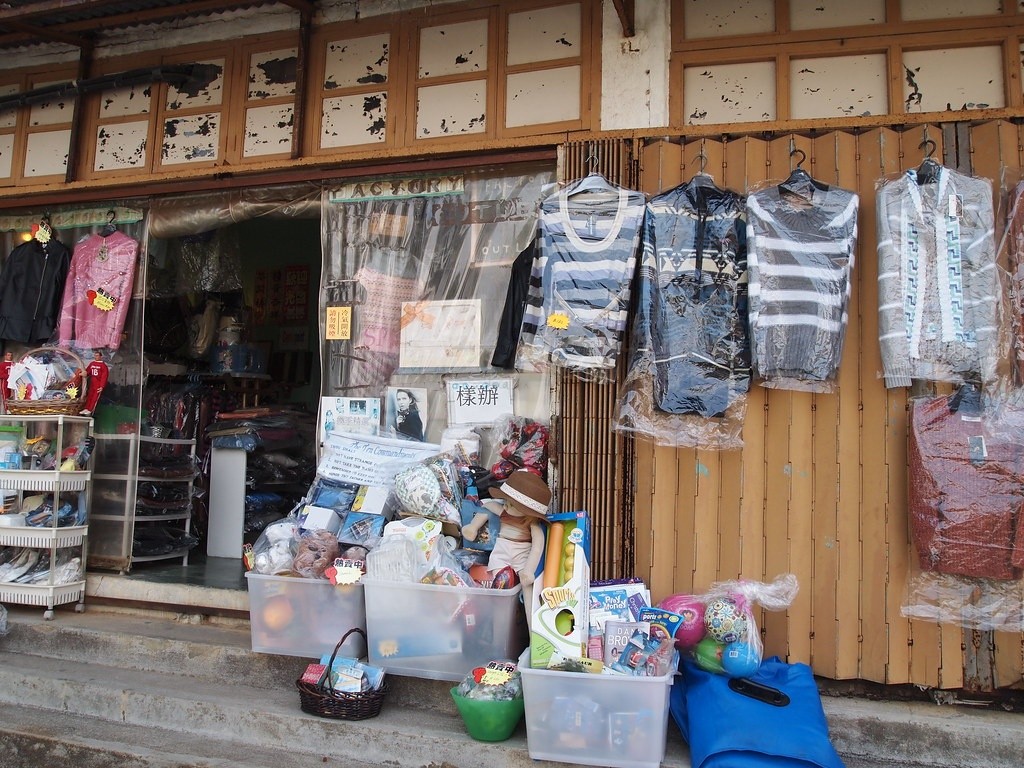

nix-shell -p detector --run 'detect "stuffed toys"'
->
[251,519,368,581]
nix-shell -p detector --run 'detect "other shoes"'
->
[0,547,81,585]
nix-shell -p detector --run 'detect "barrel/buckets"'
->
[449,687,522,742]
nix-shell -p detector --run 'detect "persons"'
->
[80,353,108,414]
[396,389,423,440]
[461,471,552,631]
[0,352,16,414]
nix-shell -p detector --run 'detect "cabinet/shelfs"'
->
[87,432,196,576]
[206,439,299,556]
[0,415,96,622]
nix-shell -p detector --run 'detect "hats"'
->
[488,471,552,524]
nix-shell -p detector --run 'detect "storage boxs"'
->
[360,572,523,682]
[318,396,380,445]
[461,499,500,550]
[245,571,366,660]
[536,511,594,665]
[352,483,396,521]
[516,644,680,768]
[338,511,389,553]
[294,505,340,538]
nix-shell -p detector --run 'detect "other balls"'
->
[655,594,760,677]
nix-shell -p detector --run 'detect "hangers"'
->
[568,154,618,196]
[685,153,726,199]
[755,149,830,204]
[97,210,116,236]
[33,215,54,240]
[186,371,207,392]
[909,137,943,178]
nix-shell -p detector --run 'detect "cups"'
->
[150,426,162,437]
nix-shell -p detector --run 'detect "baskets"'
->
[295,628,391,721]
[4,347,87,415]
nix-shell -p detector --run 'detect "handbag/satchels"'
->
[144,258,224,360]
